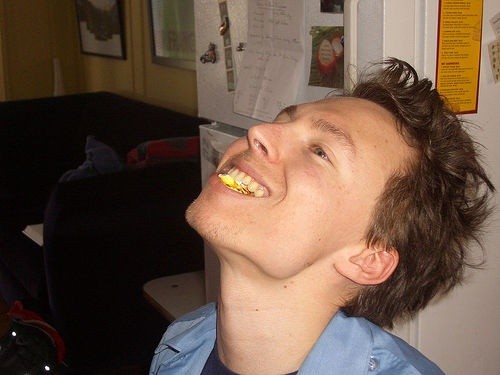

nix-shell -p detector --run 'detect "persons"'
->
[147,57,495,375]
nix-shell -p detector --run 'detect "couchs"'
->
[0,91,210,375]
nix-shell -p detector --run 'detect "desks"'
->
[142,270,206,321]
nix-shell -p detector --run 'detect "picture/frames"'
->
[76,0,126,59]
[148,0,196,70]
